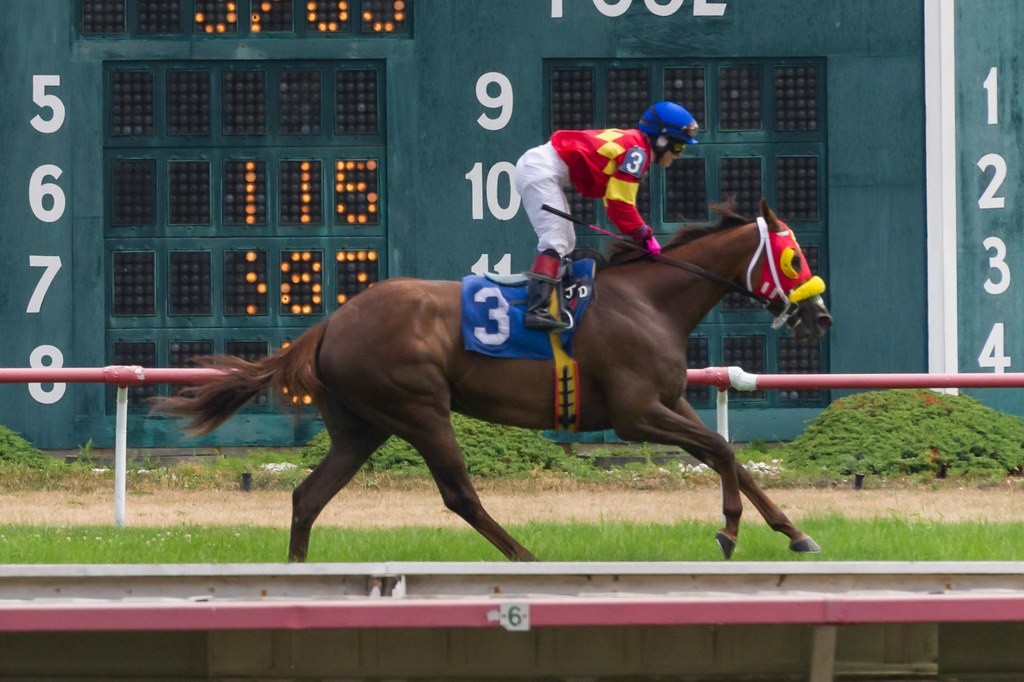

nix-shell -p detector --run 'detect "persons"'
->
[511,101,699,330]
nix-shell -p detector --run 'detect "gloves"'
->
[645,235,660,256]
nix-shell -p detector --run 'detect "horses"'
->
[148,197,833,562]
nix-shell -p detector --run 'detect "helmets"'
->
[639,102,698,144]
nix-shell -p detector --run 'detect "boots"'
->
[524,247,568,329]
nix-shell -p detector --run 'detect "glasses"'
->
[667,137,687,155]
